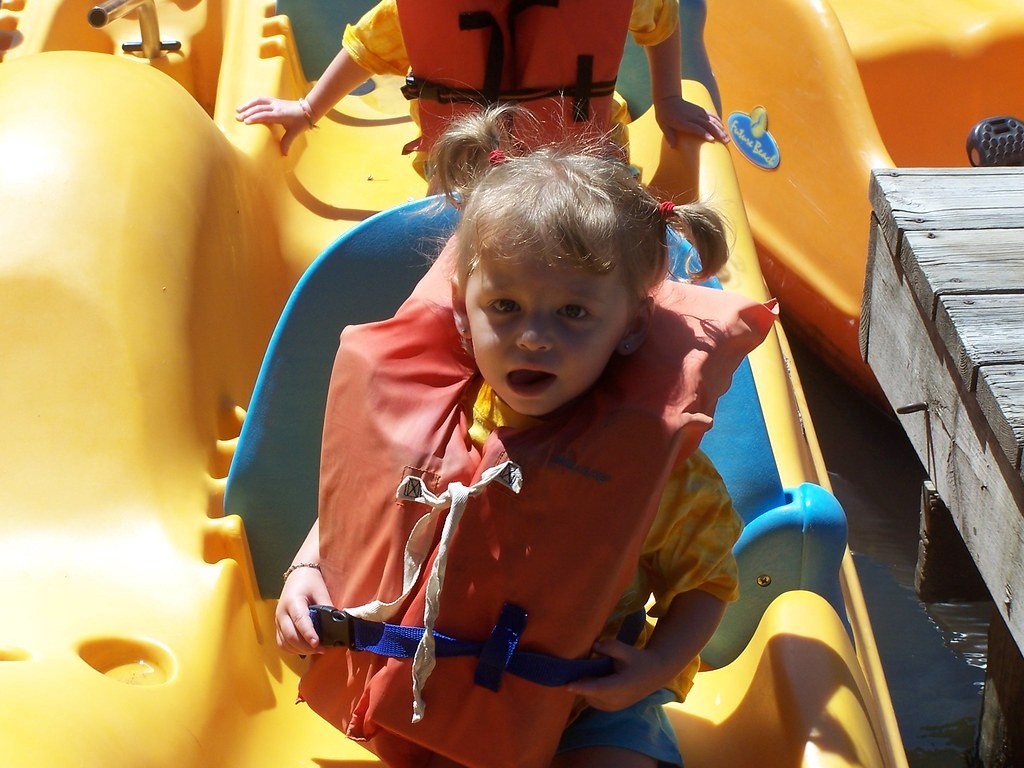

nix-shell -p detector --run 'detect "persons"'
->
[236,0,733,156]
[273,100,746,768]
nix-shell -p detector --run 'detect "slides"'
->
[202,27,797,761]
